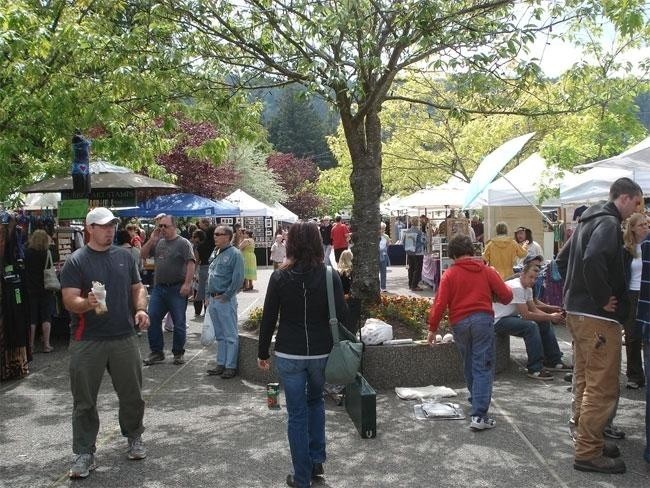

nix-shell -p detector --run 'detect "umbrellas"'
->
[462,130,554,229]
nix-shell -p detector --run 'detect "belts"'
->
[160,281,181,287]
[209,292,225,297]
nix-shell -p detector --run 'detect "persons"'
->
[395,215,486,252]
[556,177,643,473]
[427,232,513,430]
[174,220,214,317]
[314,217,353,273]
[23,230,59,352]
[568,200,625,442]
[206,225,245,379]
[623,213,649,388]
[402,218,426,291]
[118,216,146,276]
[514,226,543,271]
[380,221,393,291]
[257,219,350,488]
[481,222,530,281]
[140,215,196,365]
[493,253,570,381]
[231,223,258,290]
[635,232,650,462]
[269,225,290,270]
[60,207,151,478]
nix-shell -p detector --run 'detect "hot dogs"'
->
[92,281,108,315]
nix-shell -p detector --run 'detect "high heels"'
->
[240,285,253,292]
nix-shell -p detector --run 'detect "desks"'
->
[421,255,440,292]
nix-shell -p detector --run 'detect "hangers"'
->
[2,269,20,280]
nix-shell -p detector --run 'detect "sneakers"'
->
[143,352,165,365]
[381,288,387,292]
[574,455,627,475]
[221,370,236,379]
[206,365,224,375]
[68,451,100,479]
[470,416,497,432]
[307,462,325,475]
[564,375,644,459]
[286,473,308,488]
[545,359,574,371]
[528,369,554,381]
[412,286,423,291]
[127,435,147,461]
[173,352,184,365]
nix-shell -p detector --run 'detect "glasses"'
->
[214,233,227,236]
[159,224,169,229]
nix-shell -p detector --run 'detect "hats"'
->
[525,254,544,263]
[86,206,122,228]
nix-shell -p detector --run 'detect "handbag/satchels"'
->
[44,250,61,290]
[200,306,216,349]
[324,321,364,387]
